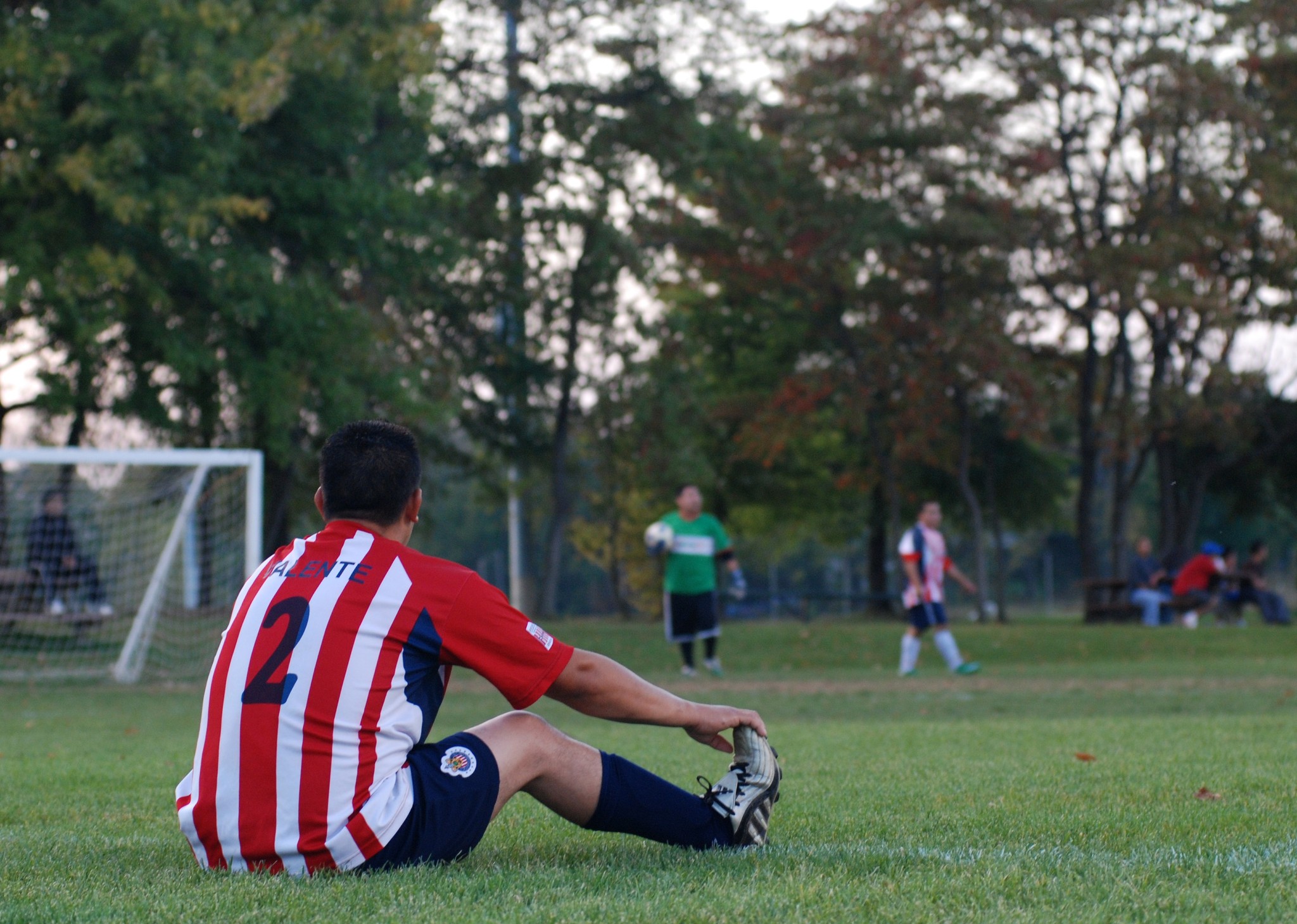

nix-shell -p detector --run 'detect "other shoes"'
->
[680,666,699,680]
[903,669,920,678]
[954,660,982,675]
[706,661,726,678]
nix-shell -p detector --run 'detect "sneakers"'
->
[699,723,783,848]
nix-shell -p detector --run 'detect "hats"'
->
[1202,540,1224,554]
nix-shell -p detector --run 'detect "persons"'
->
[1129,537,1173,626]
[897,501,981,677]
[648,482,746,676]
[1238,541,1291,626]
[1212,545,1248,628]
[175,422,783,883]
[26,487,114,618]
[1174,539,1226,627]
